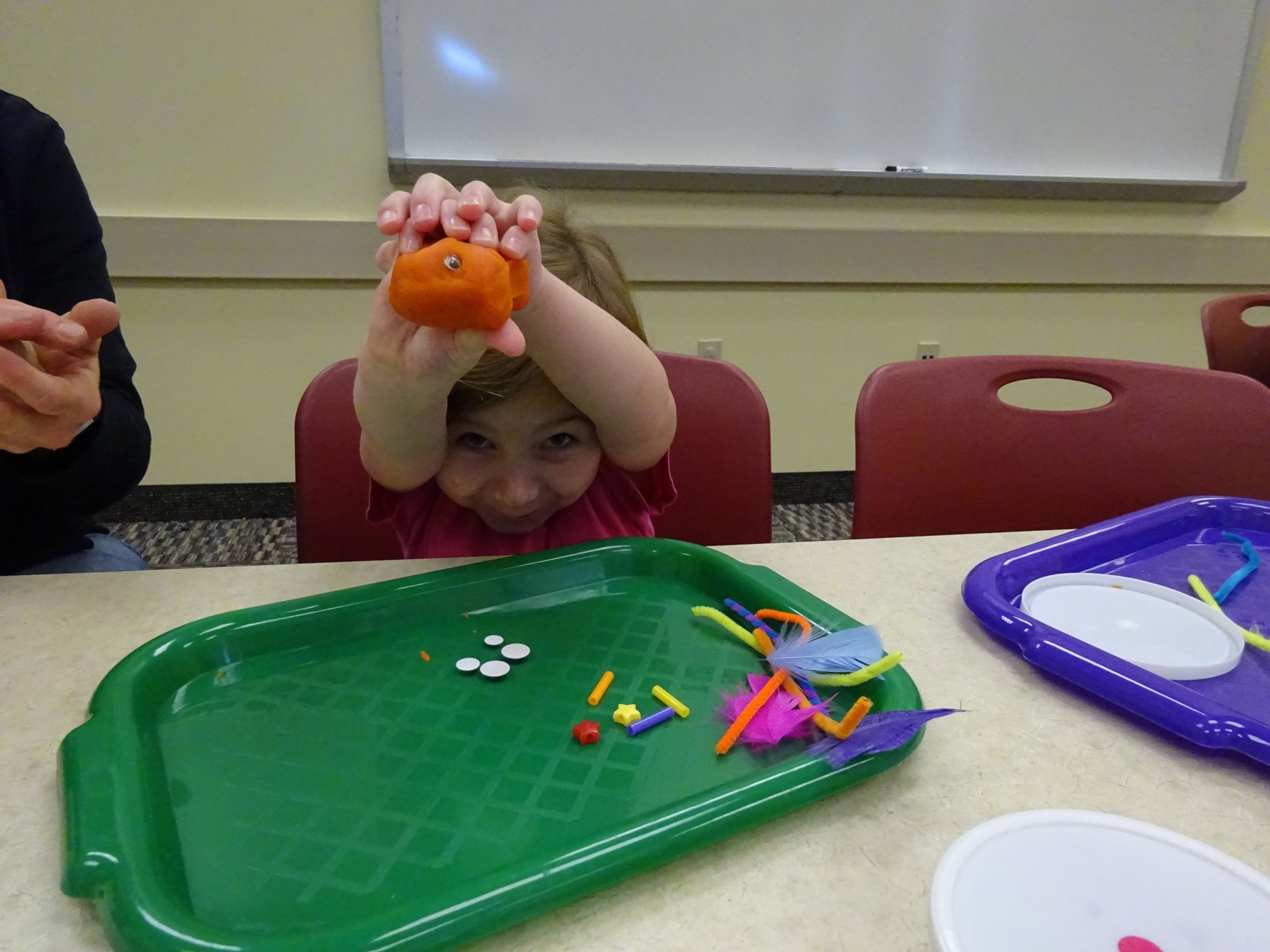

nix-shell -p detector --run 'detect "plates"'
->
[930,809,1270,952]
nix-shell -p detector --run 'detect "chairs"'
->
[854,355,1270,540]
[1202,293,1270,387]
[294,351,772,561]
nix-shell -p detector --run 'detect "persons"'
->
[353,173,677,566]
[0,89,152,576]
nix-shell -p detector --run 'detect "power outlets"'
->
[699,339,723,360]
[917,344,940,361]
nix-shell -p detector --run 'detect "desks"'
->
[0,529,1270,952]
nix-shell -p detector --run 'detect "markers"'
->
[886,166,928,173]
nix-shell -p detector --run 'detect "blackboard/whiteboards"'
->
[378,0,1270,204]
